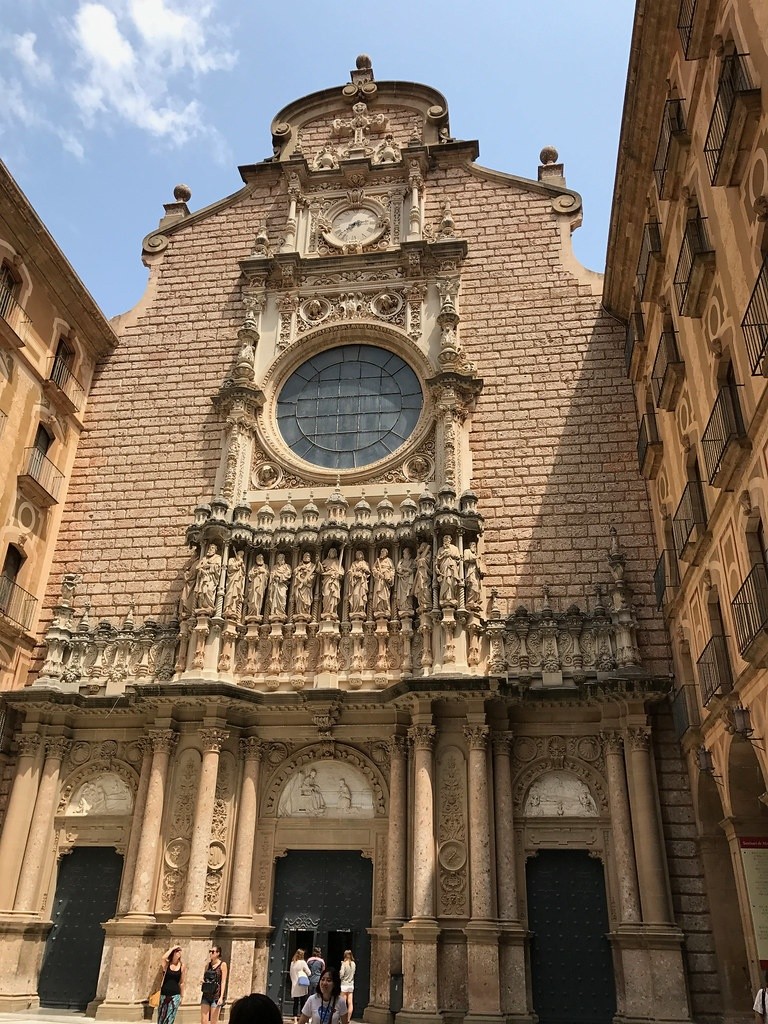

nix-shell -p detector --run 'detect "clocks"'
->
[331,207,379,242]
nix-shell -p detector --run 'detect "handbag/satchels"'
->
[298,976,310,987]
[149,991,161,1007]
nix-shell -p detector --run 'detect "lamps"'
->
[699,745,725,787]
[733,700,765,750]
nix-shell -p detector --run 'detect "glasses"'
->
[176,950,182,952]
[209,950,218,954]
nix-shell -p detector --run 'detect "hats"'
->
[162,946,183,959]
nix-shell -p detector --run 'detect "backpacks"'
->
[202,962,222,994]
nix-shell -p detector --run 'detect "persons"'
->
[157,945,186,1024]
[339,949,356,1019]
[228,993,284,1024]
[297,967,349,1024]
[201,945,227,1024]
[289,946,326,1021]
